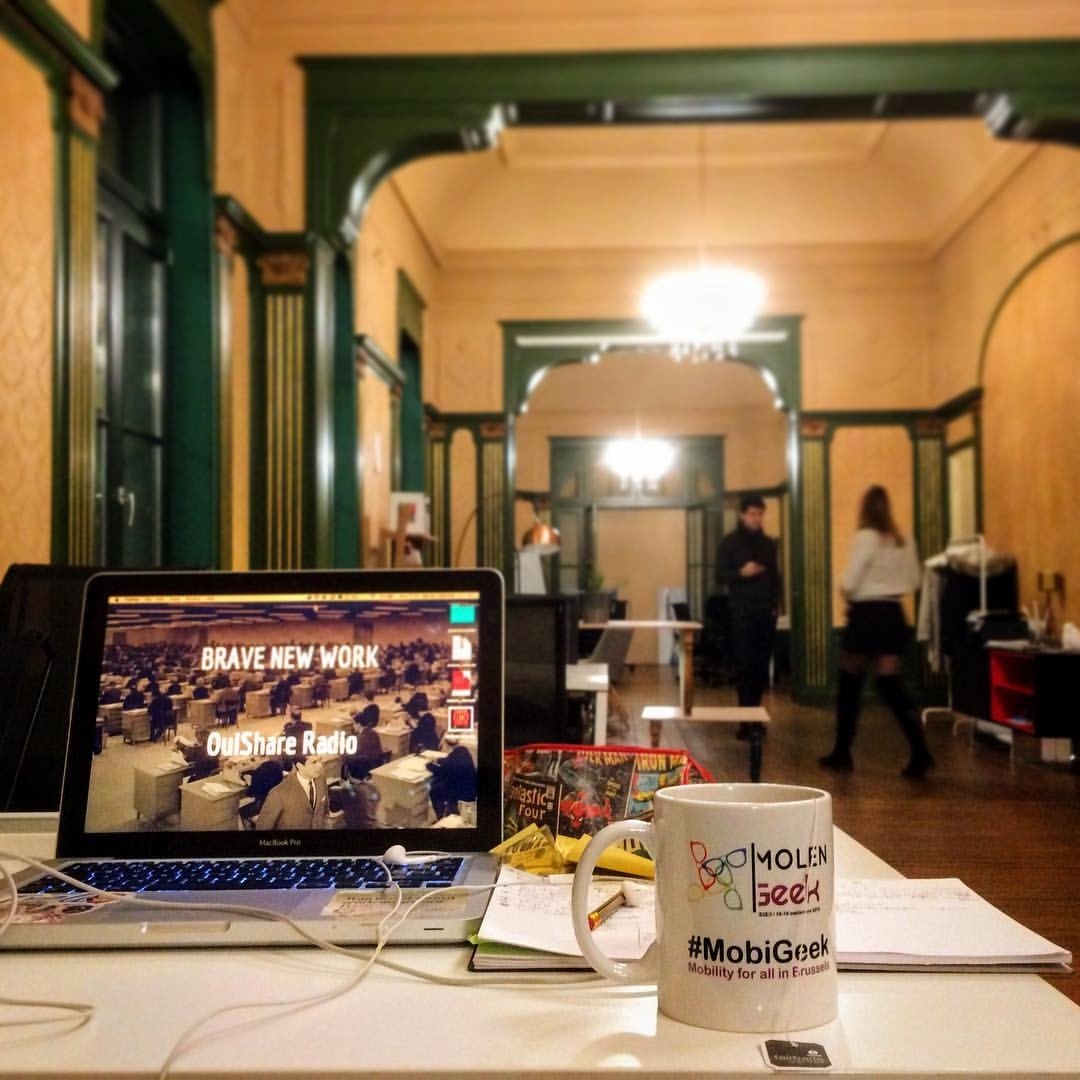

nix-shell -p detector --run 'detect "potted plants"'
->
[577,565,613,624]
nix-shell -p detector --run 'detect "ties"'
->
[309,781,314,810]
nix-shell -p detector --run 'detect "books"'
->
[468,861,1075,976]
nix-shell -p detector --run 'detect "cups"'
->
[569,774,843,1037]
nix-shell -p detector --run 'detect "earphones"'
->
[381,840,446,867]
[620,876,642,913]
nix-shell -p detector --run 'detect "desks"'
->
[576,616,704,715]
[641,703,771,783]
[0,811,1080,1079]
[567,663,611,747]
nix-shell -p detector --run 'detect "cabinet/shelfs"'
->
[985,641,1080,735]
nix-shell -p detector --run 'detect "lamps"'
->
[453,496,563,569]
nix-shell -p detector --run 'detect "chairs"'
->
[505,594,574,750]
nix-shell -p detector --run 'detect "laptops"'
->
[0,564,508,952]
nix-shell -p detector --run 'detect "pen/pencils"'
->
[587,889,626,931]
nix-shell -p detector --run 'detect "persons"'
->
[711,495,785,739]
[84,589,494,845]
[816,483,939,780]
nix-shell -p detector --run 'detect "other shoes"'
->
[736,725,766,739]
[901,761,934,777]
[820,752,853,773]
